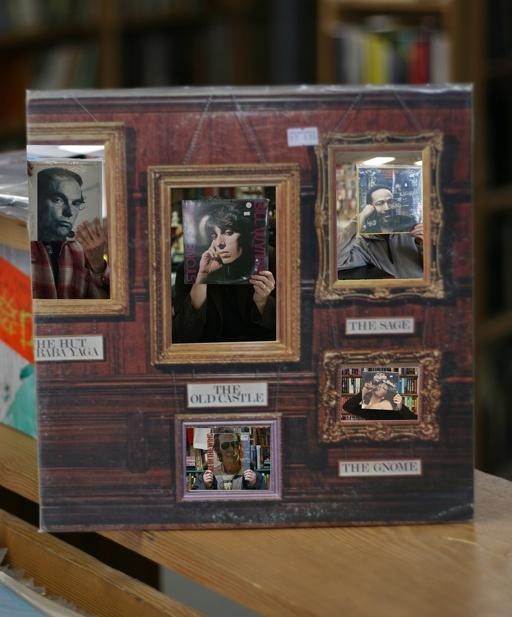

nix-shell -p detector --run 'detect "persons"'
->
[360,188,415,229]
[337,211,424,278]
[195,208,263,281]
[31,164,107,301]
[169,202,272,341]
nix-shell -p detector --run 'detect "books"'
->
[341,368,418,420]
[184,425,270,491]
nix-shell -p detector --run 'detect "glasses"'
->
[48,191,86,211]
[221,441,239,449]
